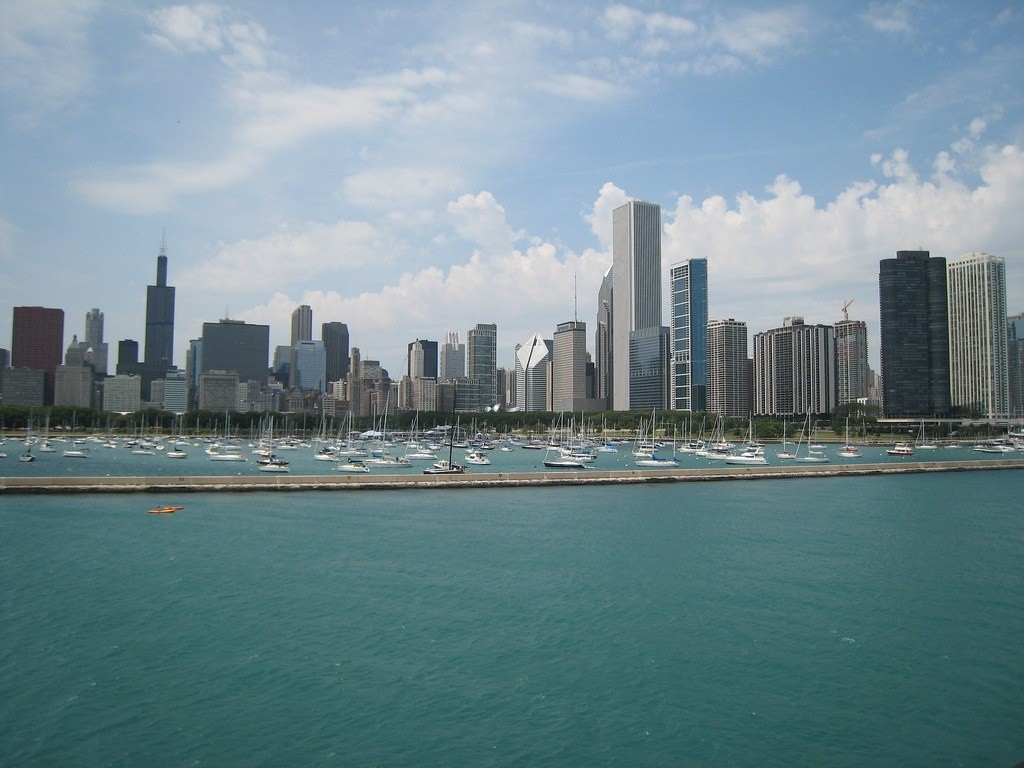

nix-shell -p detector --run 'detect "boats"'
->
[163,507,184,510]
[148,509,174,513]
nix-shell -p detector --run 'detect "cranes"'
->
[842,299,854,320]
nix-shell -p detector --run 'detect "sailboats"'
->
[0,407,1024,474]
